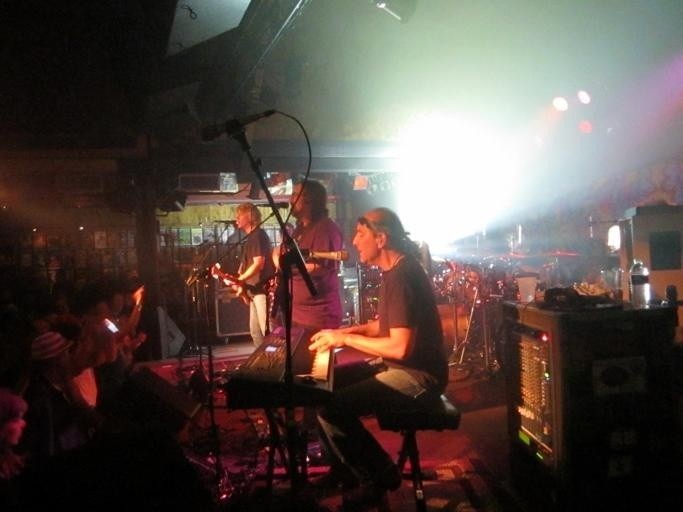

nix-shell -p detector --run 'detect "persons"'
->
[414,250,579,368]
[235,203,277,350]
[269,179,350,466]
[0,217,206,512]
[306,206,451,507]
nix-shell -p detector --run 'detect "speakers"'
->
[215,296,251,344]
[108,366,202,444]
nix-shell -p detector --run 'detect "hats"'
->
[31,330,74,360]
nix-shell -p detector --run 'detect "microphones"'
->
[202,109,273,141]
[306,250,348,261]
[216,220,235,224]
[256,202,288,208]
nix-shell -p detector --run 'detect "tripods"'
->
[196,268,265,512]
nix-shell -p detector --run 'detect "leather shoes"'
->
[343,464,401,512]
[312,465,360,490]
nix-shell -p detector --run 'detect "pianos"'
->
[222,321,336,410]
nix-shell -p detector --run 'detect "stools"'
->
[380,395,461,510]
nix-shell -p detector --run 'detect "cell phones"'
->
[102,317,120,334]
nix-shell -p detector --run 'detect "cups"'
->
[514,270,538,306]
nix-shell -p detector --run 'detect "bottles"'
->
[627,257,653,309]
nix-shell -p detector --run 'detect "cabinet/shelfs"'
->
[501,294,679,511]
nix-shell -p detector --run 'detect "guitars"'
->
[211,262,250,306]
